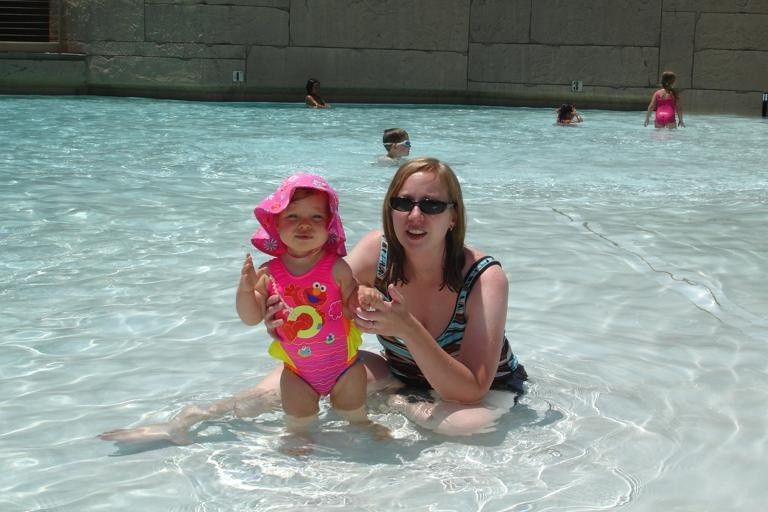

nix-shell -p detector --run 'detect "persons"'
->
[644,71,686,129]
[235,171,386,414]
[306,79,329,109]
[377,128,411,165]
[556,104,583,126]
[266,158,528,428]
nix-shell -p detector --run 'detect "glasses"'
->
[383,140,411,147]
[390,197,456,215]
